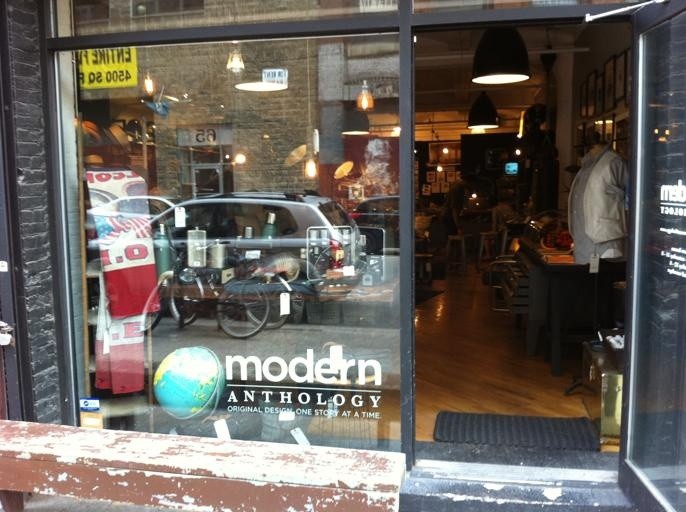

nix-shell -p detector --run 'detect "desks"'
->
[509,236,614,357]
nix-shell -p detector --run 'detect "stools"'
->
[477,232,501,274]
[444,235,467,273]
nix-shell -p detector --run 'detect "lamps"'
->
[226,42,245,76]
[357,80,374,112]
[466,92,500,130]
[471,24,529,85]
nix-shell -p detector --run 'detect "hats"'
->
[104,125,129,150]
[81,120,103,147]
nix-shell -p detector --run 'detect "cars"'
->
[348,195,400,255]
[83,195,190,245]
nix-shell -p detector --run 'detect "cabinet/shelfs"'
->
[79,141,220,433]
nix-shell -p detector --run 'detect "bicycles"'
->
[167,228,294,330]
[137,232,271,339]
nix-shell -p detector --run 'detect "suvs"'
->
[83,187,369,323]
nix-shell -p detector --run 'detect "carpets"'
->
[434,410,599,453]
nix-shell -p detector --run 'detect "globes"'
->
[151,343,226,436]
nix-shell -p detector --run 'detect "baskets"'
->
[342,302,381,327]
[306,301,340,326]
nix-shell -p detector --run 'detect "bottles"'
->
[187,227,206,268]
[154,224,170,279]
[259,211,277,250]
[209,224,256,269]
[308,228,353,281]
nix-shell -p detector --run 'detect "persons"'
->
[419,188,507,264]
[567,142,629,385]
[358,139,392,197]
